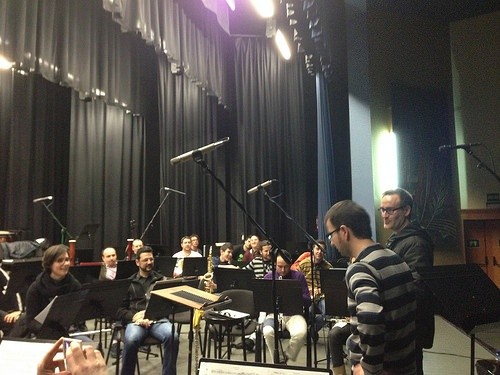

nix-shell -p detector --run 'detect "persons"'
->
[235,240,275,352]
[119,245,179,375]
[297,240,335,343]
[172,235,203,278]
[0,245,31,338]
[380,187,435,375]
[232,235,253,265]
[290,240,313,271]
[93,247,130,280]
[329,317,350,375]
[189,234,202,255]
[243,234,260,265]
[36,337,108,375]
[262,250,312,365]
[324,200,419,375]
[130,239,147,259]
[212,243,236,267]
[27,245,92,340]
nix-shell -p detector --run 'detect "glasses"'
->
[326,227,341,240]
[55,256,70,266]
[378,206,404,215]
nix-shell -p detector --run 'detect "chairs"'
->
[0,228,392,375]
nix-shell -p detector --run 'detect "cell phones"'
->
[63,337,82,371]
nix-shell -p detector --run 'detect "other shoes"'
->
[240,339,255,349]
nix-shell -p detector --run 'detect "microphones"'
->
[164,187,185,195]
[33,196,52,202]
[439,142,480,153]
[477,159,486,168]
[247,179,278,195]
[170,140,229,166]
